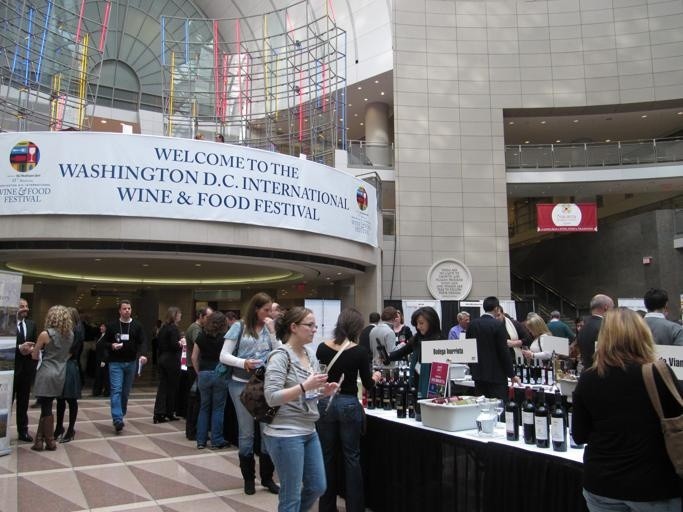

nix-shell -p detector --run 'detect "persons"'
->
[153,307,187,424]
[448,311,470,340]
[225,312,239,329]
[220,293,283,495]
[31,306,74,451]
[572,306,683,512]
[546,311,576,345]
[370,307,406,367]
[390,310,414,349]
[466,296,524,423]
[186,308,212,441]
[191,311,231,449]
[11,299,38,443]
[100,300,147,432]
[522,315,552,366]
[632,310,683,330]
[569,318,586,375]
[496,306,534,364]
[578,294,613,370]
[360,312,379,362]
[214,134,224,142]
[93,323,111,396]
[379,306,448,364]
[316,306,381,512]
[54,307,85,443]
[642,289,682,346]
[194,132,204,140]
[264,307,340,512]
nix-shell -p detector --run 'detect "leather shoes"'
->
[113,417,124,432]
[18,432,33,441]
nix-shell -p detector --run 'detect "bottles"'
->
[375,384,384,409]
[541,360,548,386]
[550,390,568,452]
[367,389,375,409]
[535,359,541,385]
[392,371,399,408]
[529,358,537,385]
[523,356,531,384]
[547,358,554,385]
[534,387,550,449]
[504,385,519,441]
[415,391,423,423]
[394,375,407,419]
[514,356,523,382]
[407,375,416,418]
[381,373,391,409]
[521,386,537,444]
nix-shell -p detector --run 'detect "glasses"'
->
[297,322,319,331]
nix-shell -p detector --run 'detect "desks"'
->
[358,399,585,512]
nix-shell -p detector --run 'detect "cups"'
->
[399,335,405,343]
[522,345,528,350]
[116,333,121,343]
[317,364,327,394]
[494,399,504,427]
[373,359,383,383]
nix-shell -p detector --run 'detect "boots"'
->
[31,418,45,451]
[258,456,282,495]
[42,411,56,450]
[238,449,256,495]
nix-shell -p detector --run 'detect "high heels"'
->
[153,415,170,424]
[53,428,65,439]
[166,412,180,421]
[60,430,76,443]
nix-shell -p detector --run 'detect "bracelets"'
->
[299,383,305,394]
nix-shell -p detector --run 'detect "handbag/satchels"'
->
[239,347,291,425]
[637,354,683,475]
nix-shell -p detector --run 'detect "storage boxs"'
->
[560,376,578,398]
[418,395,501,431]
[447,364,466,382]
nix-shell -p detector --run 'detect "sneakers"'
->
[209,442,233,451]
[194,440,208,452]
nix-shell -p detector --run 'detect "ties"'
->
[17,321,24,353]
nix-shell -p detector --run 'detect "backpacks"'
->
[214,312,244,380]
